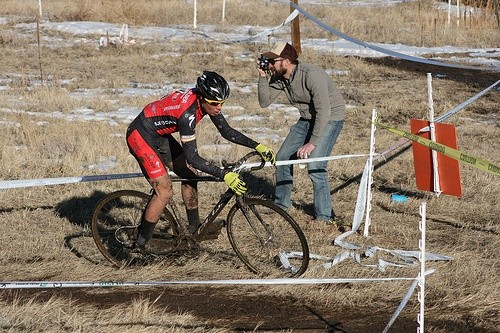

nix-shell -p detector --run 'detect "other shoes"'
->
[133,239,174,255]
[204,218,225,234]
[260,208,275,214]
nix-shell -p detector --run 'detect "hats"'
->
[260,42,298,61]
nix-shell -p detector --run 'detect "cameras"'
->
[261,55,269,70]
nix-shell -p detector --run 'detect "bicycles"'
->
[91,149,310,279]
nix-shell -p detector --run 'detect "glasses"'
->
[204,98,225,106]
[268,58,283,65]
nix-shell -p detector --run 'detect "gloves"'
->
[223,171,247,195]
[255,143,276,166]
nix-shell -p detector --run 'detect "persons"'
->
[126,71,278,253]
[258,41,345,224]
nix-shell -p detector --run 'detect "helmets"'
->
[197,70,230,101]
